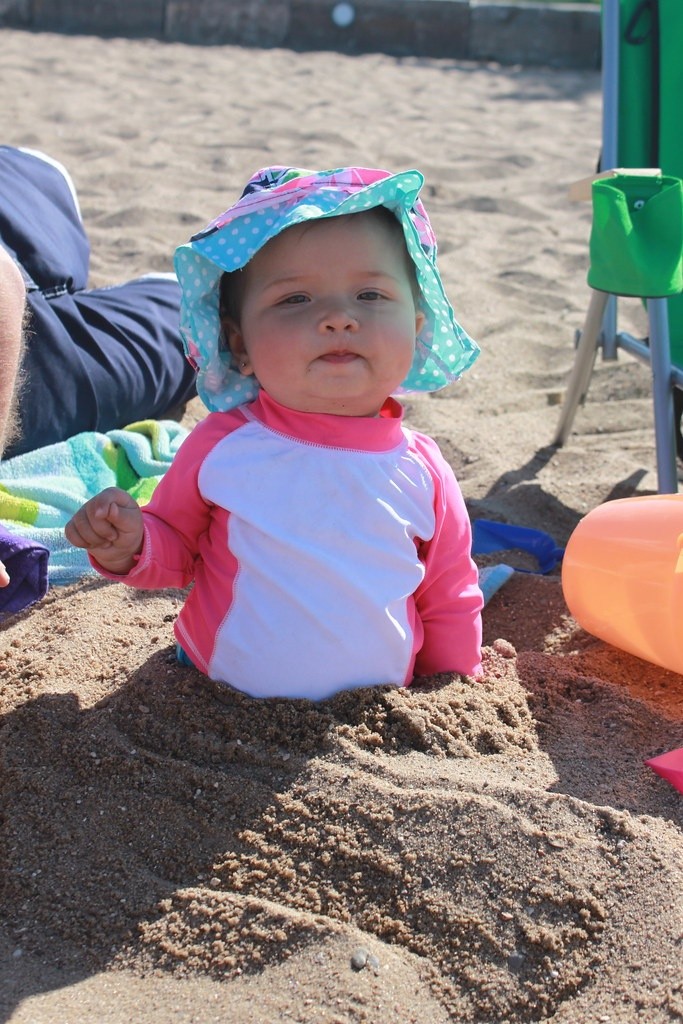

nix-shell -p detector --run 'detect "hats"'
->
[173,165,483,414]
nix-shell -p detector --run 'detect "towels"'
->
[0,419,513,611]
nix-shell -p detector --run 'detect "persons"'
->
[65,162,486,700]
[0,143,200,613]
